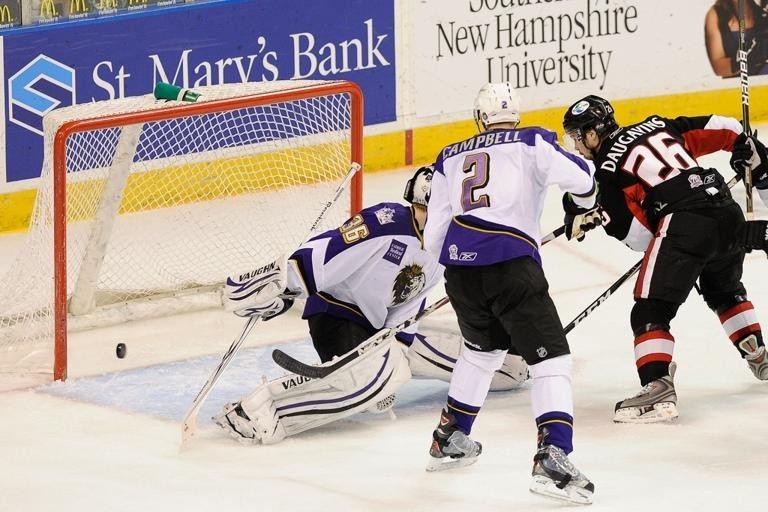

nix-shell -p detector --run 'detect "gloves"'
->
[728,131,768,190]
[561,191,612,242]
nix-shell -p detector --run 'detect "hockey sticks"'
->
[272,225,564,379]
[179,161,362,465]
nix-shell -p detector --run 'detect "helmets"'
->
[472,80,522,134]
[562,94,618,150]
[403,161,436,207]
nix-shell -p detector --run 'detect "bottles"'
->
[154,81,207,102]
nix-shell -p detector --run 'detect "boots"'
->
[737,334,768,382]
[428,406,483,461]
[532,424,596,495]
[614,359,677,416]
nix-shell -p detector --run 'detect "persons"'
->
[421,80,596,494]
[705,0,768,78]
[562,94,768,412]
[207,163,530,445]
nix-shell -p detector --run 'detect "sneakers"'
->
[223,401,257,441]
